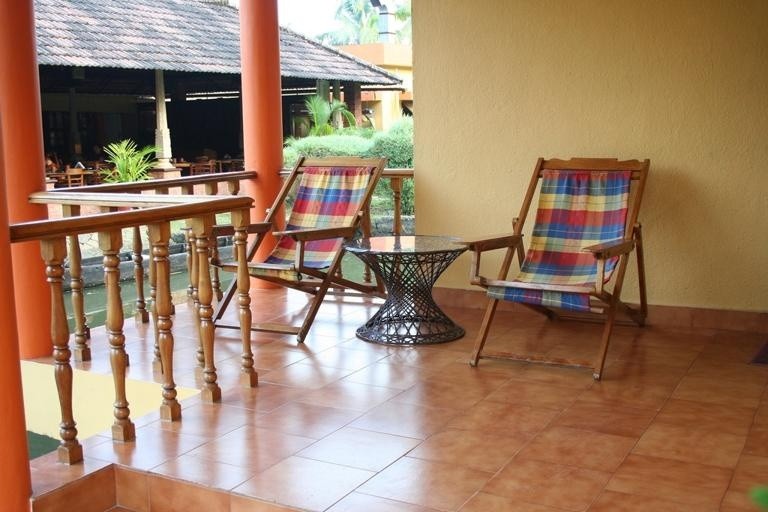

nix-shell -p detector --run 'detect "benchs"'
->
[47,153,246,187]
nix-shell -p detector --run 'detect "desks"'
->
[343,235,469,346]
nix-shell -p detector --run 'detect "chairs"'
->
[453,156,650,382]
[210,156,400,341]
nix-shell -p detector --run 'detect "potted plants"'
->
[101,141,162,210]
